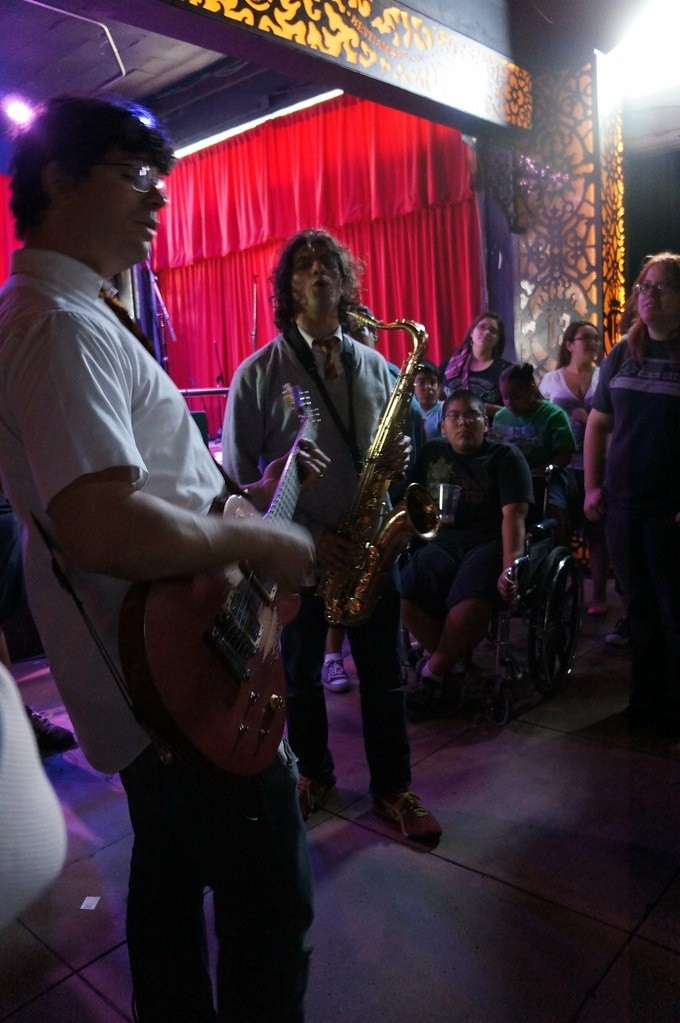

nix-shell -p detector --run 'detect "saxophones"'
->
[323,305,440,629]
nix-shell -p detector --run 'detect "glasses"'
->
[87,161,166,199]
[444,410,483,423]
[636,284,680,295]
[574,334,602,343]
[477,323,499,335]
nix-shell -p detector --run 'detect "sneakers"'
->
[27,706,74,747]
[297,772,336,820]
[606,614,630,645]
[320,659,348,692]
[370,789,443,841]
[405,657,469,720]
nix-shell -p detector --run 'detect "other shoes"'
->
[588,597,608,614]
[625,705,680,739]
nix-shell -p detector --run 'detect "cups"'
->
[435,484,462,523]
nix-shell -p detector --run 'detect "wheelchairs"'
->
[403,464,583,727]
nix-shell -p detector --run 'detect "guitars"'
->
[115,383,323,779]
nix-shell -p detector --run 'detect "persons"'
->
[316,249,678,754]
[0,93,318,1021]
[0,625,76,760]
[220,227,444,850]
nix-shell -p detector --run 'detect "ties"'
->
[320,339,338,380]
[99,286,156,359]
